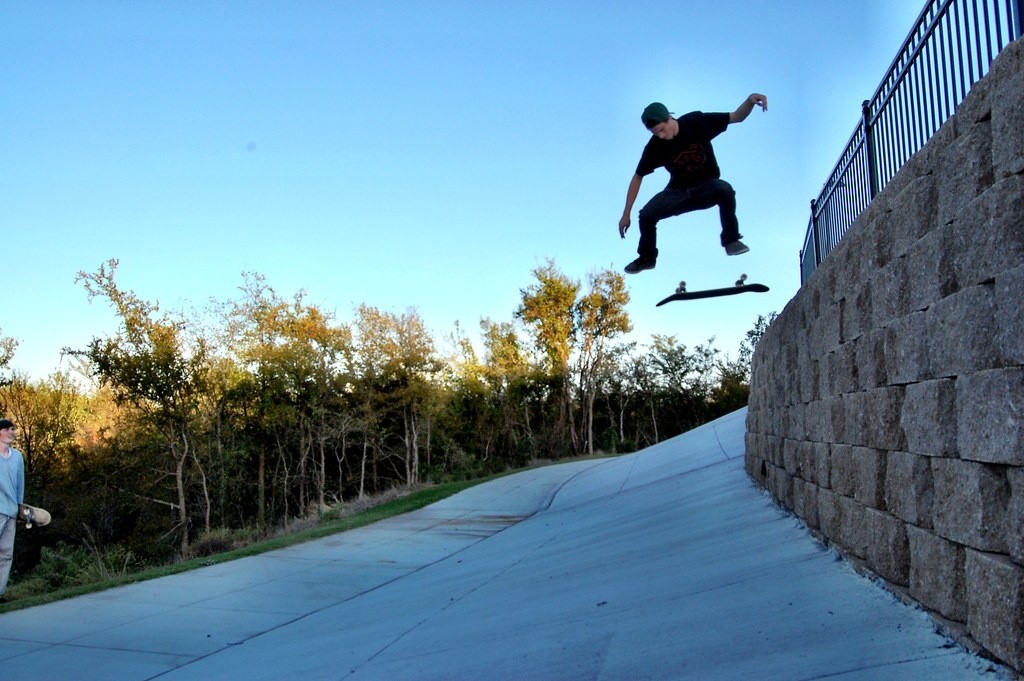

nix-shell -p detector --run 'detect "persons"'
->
[617,92,766,274]
[0,419,26,603]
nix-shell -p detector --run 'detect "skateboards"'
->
[656,274,769,306]
[17,503,51,529]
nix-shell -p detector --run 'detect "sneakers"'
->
[625,256,657,274]
[726,241,749,256]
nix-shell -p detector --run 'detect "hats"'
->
[641,102,675,129]
